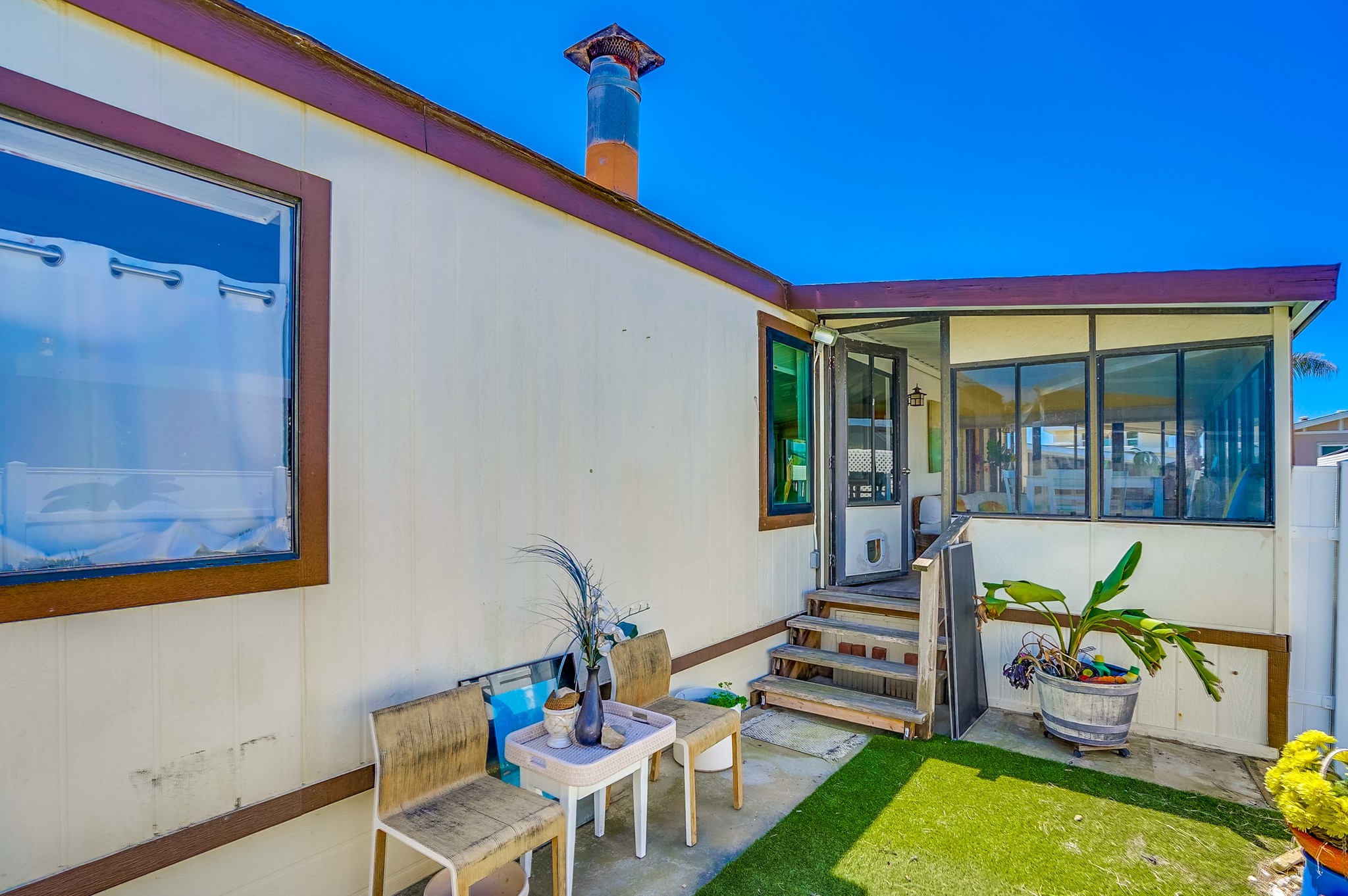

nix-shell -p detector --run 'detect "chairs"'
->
[606,629,742,847]
[367,682,567,896]
[1003,468,1199,518]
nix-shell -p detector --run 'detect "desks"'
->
[505,700,676,896]
[1023,475,1165,516]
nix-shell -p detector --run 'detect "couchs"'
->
[914,491,1009,558]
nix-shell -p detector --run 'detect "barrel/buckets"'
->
[424,859,529,896]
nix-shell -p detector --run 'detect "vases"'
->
[1035,659,1142,746]
[576,665,604,746]
[1286,820,1348,895]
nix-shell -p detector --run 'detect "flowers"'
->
[1264,729,1348,852]
[974,541,1224,702]
[503,534,650,719]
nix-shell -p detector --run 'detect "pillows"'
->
[955,494,966,512]
[979,501,1008,512]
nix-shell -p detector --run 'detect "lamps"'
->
[908,383,927,406]
[810,324,839,363]
[1000,427,1011,433]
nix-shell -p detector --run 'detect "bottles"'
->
[1092,654,1110,677]
[1123,665,1140,683]
